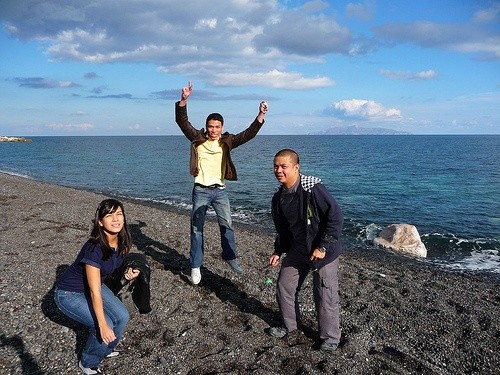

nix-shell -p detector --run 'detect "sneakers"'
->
[320,340,337,351]
[270,327,288,337]
[190,267,201,284]
[79,360,102,375]
[221,257,243,273]
[104,352,119,357]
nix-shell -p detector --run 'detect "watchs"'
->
[319,247,327,253]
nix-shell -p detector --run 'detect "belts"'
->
[196,184,222,189]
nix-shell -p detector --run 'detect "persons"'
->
[269,149,344,351]
[175,80,268,284]
[54,198,142,374]
